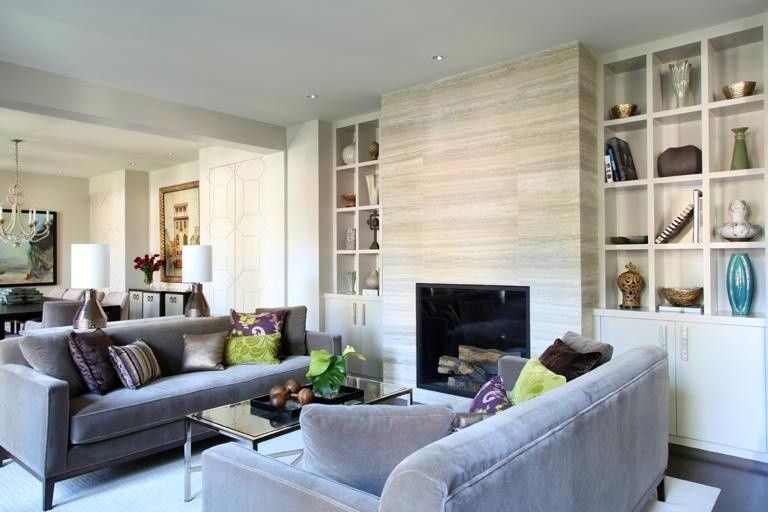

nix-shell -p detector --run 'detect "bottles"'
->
[344,227,356,249]
[366,269,379,289]
[725,252,754,317]
[716,198,763,242]
[341,136,355,164]
[729,126,751,170]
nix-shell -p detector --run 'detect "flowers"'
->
[133,255,166,283]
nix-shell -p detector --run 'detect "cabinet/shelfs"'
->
[588,14,768,461]
[126,289,191,322]
[326,118,378,373]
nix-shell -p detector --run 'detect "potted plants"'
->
[304,342,366,397]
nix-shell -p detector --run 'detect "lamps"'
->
[0,137,51,249]
[180,242,213,319]
[72,245,110,329]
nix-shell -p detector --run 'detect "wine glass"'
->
[344,271,356,295]
[340,193,355,207]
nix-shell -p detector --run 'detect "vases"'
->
[143,271,153,285]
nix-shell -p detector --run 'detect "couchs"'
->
[0,304,343,510]
[200,342,669,511]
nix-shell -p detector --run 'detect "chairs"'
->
[22,300,85,333]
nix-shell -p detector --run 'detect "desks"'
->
[0,297,121,339]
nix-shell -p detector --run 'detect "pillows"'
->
[21,328,161,395]
[175,307,289,372]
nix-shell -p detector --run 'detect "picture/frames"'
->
[157,180,205,283]
[0,210,57,288]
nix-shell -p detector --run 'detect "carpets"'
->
[0,430,722,512]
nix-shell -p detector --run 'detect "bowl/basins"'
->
[610,103,637,119]
[661,285,703,306]
[722,79,756,99]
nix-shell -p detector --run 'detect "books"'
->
[658,302,703,314]
[693,188,702,243]
[697,196,703,243]
[605,136,639,183]
[654,201,693,243]
